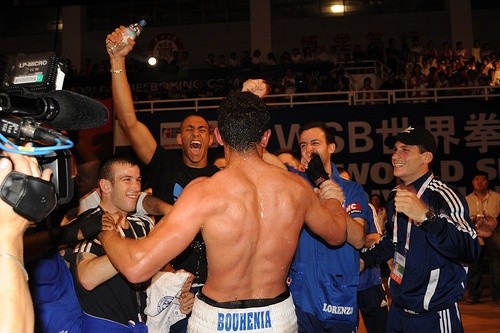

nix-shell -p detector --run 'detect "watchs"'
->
[415,210,436,226]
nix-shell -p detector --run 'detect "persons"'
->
[203,48,265,69]
[125,49,192,74]
[268,44,371,102]
[97,93,347,333]
[213,79,369,333]
[377,37,500,104]
[1,139,195,333]
[108,26,223,295]
[359,127,478,333]
[371,194,388,234]
[358,76,377,105]
[463,170,500,306]
[335,167,390,333]
[63,57,111,75]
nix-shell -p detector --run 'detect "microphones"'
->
[0,89,108,129]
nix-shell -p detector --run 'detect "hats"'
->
[383,126,437,154]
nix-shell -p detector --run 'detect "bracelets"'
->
[109,68,126,74]
[0,252,29,281]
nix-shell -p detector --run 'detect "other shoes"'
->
[495,297,500,306]
[466,297,478,304]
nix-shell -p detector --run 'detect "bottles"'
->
[106,20,146,57]
[475,209,482,224]
